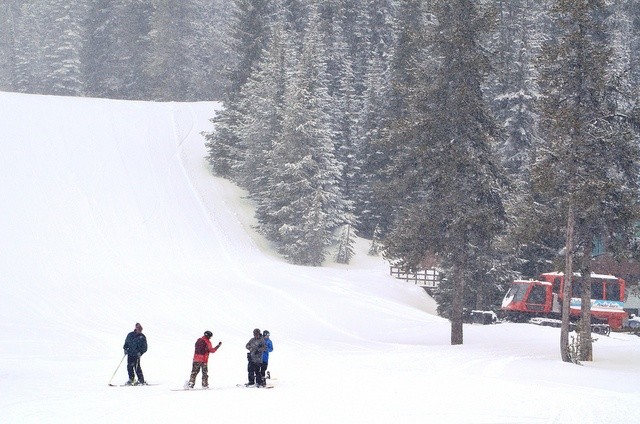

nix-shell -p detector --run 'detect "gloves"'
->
[124,350,127,354]
[137,352,141,356]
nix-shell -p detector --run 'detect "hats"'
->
[263,331,269,336]
[136,323,142,330]
[253,329,260,336]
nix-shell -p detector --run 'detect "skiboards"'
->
[109,384,159,386]
[172,388,212,391]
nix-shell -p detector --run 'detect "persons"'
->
[123,322,148,386]
[259,330,273,376]
[629,313,640,321]
[245,328,268,387]
[187,331,223,389]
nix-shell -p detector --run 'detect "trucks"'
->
[499,270,626,332]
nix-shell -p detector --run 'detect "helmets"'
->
[204,331,212,336]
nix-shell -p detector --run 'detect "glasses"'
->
[265,333,270,336]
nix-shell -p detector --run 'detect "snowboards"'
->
[236,384,274,389]
